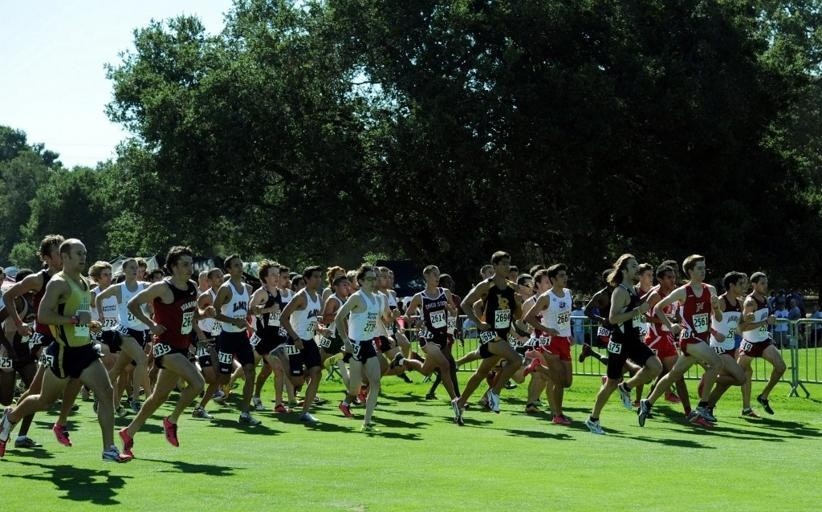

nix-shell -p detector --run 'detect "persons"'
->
[392,250,822,434]
[0,235,409,464]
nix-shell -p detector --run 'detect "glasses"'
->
[362,275,377,281]
